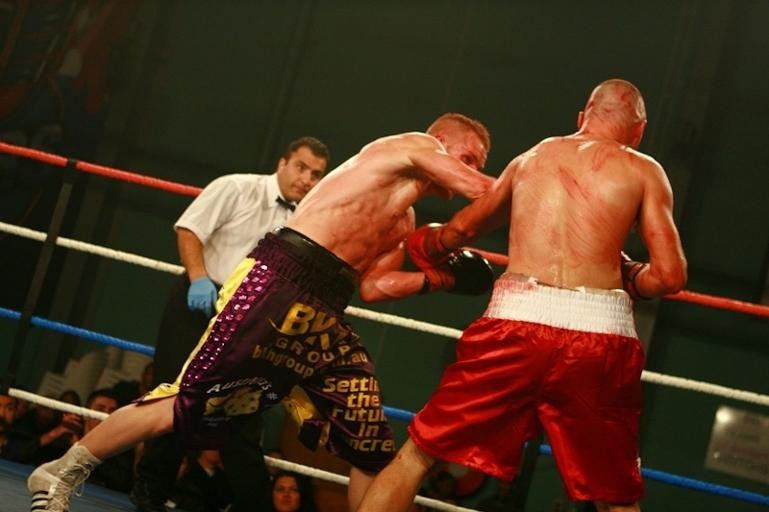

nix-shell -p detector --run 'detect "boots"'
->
[28,441,101,512]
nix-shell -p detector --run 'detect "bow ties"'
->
[276,197,296,211]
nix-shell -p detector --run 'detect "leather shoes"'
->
[130,481,170,512]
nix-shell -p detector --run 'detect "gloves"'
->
[621,252,648,301]
[190,277,218,317]
[420,251,495,295]
[405,222,458,269]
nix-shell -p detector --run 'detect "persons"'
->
[27,112,497,512]
[129,137,331,512]
[357,79,687,512]
[0,376,148,493]
[179,451,317,512]
